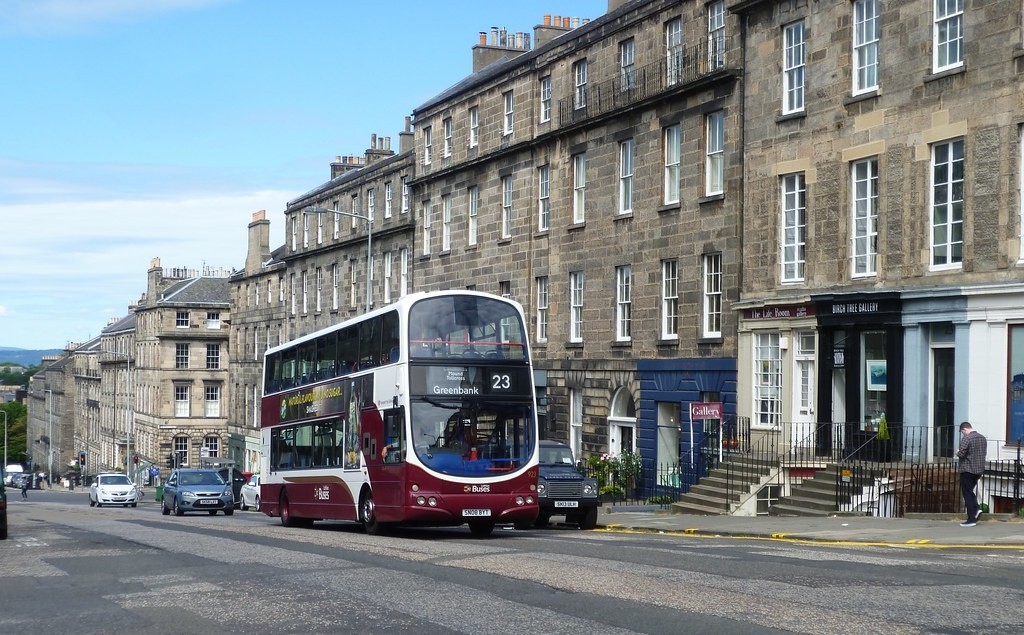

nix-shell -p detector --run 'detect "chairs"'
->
[389,346,399,363]
[412,346,435,357]
[484,349,506,359]
[269,352,390,393]
[462,349,482,358]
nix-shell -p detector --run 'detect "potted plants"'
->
[873,415,891,463]
[723,427,738,449]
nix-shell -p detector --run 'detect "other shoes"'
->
[959,520,977,527]
[974,508,983,522]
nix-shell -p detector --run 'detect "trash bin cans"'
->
[154,486,163,502]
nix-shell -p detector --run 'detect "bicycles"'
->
[135,488,143,501]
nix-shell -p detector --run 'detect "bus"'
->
[258,290,553,539]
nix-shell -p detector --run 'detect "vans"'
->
[3,465,23,486]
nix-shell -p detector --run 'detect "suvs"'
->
[481,442,601,533]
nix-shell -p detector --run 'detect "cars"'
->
[88,473,139,508]
[184,468,247,508]
[11,473,39,489]
[239,473,261,512]
[161,469,235,516]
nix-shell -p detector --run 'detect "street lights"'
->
[304,205,372,312]
[30,390,51,488]
[96,350,130,478]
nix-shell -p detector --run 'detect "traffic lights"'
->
[81,453,86,465]
[134,456,138,464]
[165,453,172,468]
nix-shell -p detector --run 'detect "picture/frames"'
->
[866,360,887,391]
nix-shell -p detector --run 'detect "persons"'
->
[954,421,987,528]
[381,421,430,459]
